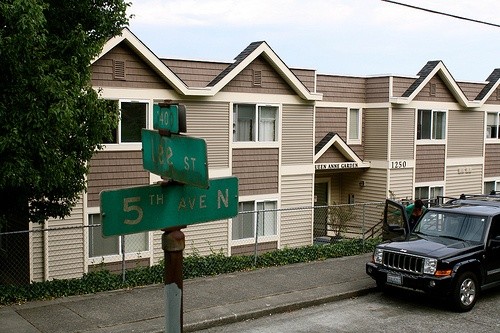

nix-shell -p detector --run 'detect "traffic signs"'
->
[98,176,238,239]
[140,127,212,190]
[152,102,181,135]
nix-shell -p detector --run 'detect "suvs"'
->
[365,193,500,312]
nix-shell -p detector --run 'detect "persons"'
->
[384,200,428,233]
